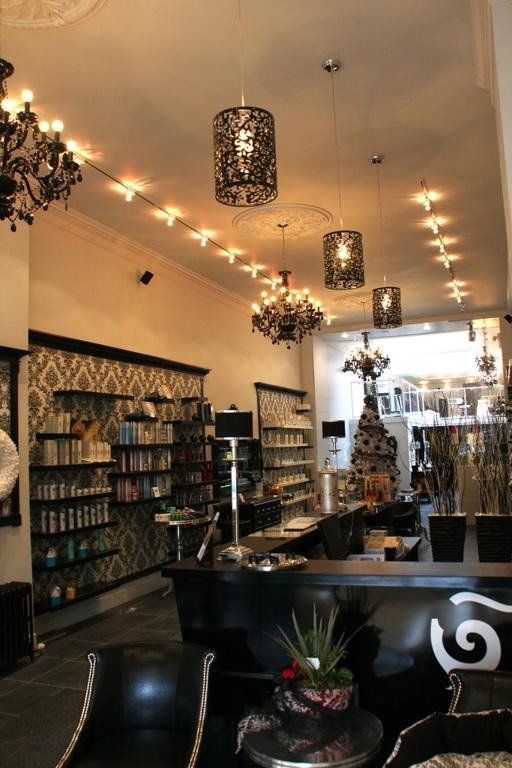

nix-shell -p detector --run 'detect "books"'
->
[343,553,385,562]
[364,536,410,560]
[284,517,322,530]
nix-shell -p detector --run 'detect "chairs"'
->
[383,666,512,768]
[361,501,419,535]
[58,641,215,768]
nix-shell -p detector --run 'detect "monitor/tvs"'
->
[317,513,347,560]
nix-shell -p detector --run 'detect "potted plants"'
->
[467,389,512,562]
[394,392,472,563]
[263,602,371,717]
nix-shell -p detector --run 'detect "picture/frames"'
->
[0,344,32,528]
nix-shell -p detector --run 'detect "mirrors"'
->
[215,437,263,498]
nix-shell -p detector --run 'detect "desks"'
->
[237,703,384,768]
[154,512,212,600]
[364,534,422,561]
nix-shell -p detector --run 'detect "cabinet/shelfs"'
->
[254,382,315,522]
[28,327,214,618]
[218,495,282,542]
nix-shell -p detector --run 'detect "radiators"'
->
[0,581,37,673]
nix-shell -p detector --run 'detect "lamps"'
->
[343,299,390,385]
[247,223,324,347]
[364,475,392,511]
[0,54,88,232]
[320,58,368,291]
[321,419,346,474]
[214,403,254,542]
[370,154,403,329]
[211,0,280,207]
